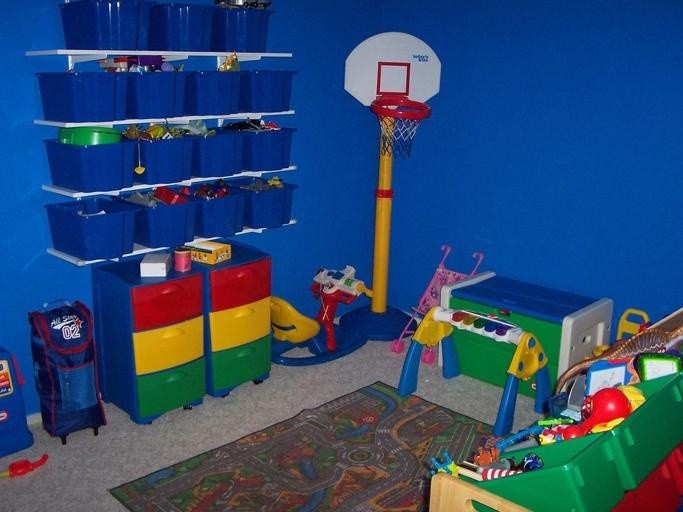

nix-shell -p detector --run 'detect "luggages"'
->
[28,299,108,445]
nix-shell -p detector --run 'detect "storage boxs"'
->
[42,126,293,194]
[58,1,276,54]
[33,68,294,123]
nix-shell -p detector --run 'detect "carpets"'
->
[108,380,513,512]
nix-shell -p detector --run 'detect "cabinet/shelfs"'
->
[25,49,293,71]
[40,164,296,200]
[32,109,296,128]
[46,218,297,268]
[92,239,271,427]
